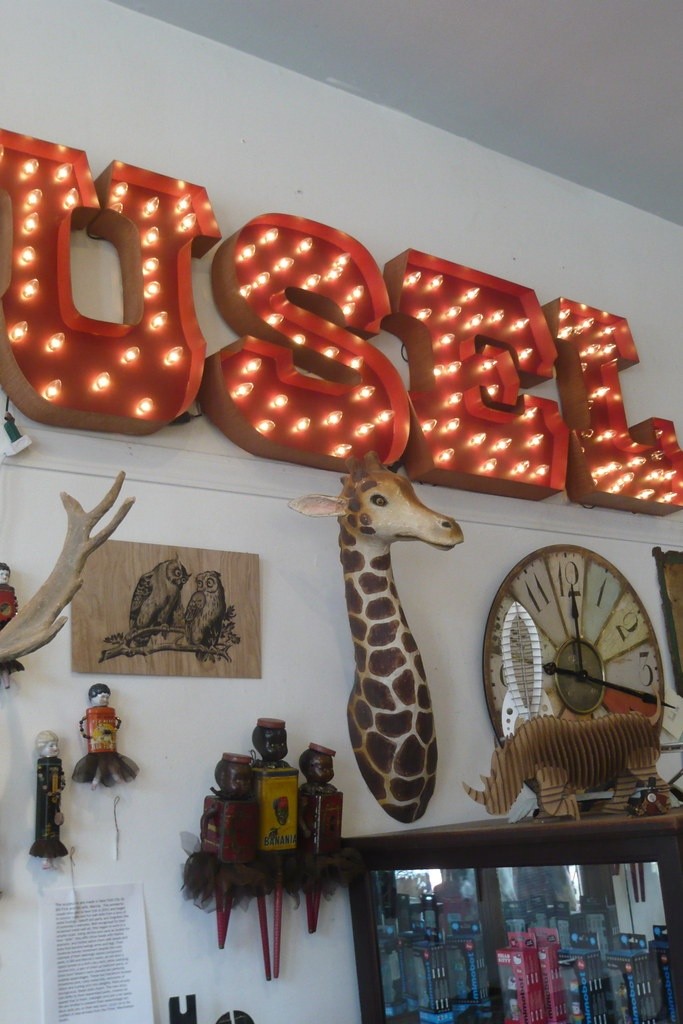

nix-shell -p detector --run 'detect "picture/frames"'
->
[652,547,683,699]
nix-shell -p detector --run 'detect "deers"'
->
[284,449,466,825]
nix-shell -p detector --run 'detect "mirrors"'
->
[371,862,666,1019]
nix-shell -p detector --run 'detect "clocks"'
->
[481,545,665,794]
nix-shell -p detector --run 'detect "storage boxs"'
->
[503,894,621,961]
[496,927,677,1024]
[377,921,492,1024]
[395,893,483,943]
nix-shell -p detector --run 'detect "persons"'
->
[28,729,68,870]
[71,683,140,791]
[0,563,26,688]
[178,717,368,982]
